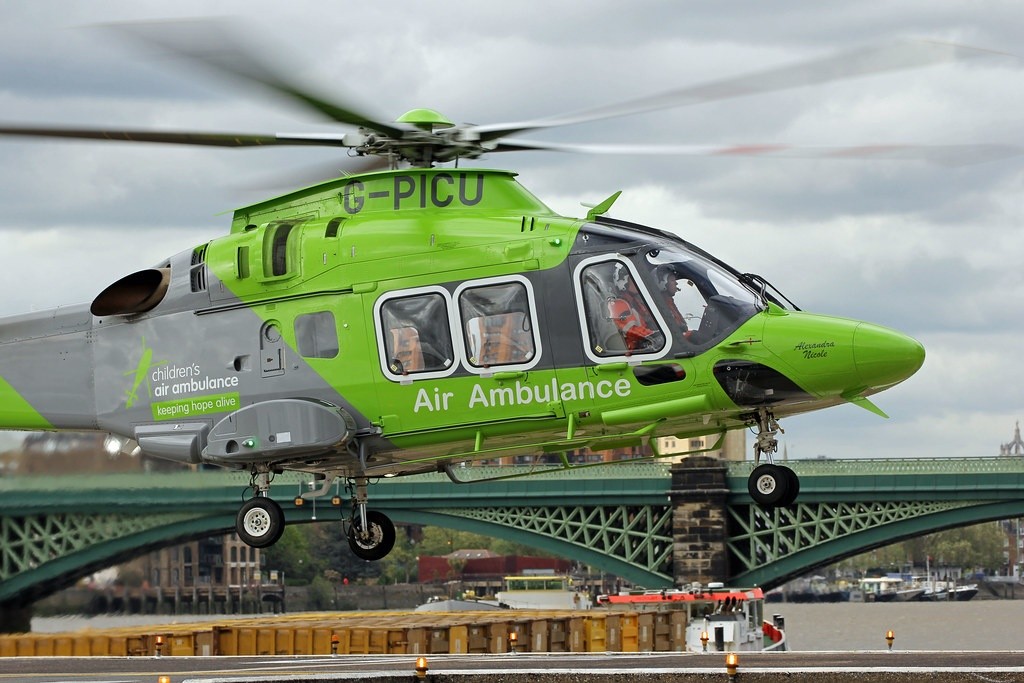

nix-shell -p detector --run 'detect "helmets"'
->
[613,263,630,292]
[651,264,676,293]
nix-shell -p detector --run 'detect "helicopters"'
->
[0,6,1022,563]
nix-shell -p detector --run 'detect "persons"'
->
[608,263,660,354]
[648,263,694,339]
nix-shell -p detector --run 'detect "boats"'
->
[383,569,1022,656]
